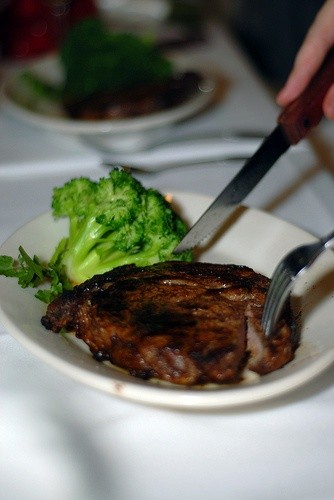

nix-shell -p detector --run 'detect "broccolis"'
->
[52,167,193,286]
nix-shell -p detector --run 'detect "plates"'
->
[0,189,334,409]
[1,56,220,134]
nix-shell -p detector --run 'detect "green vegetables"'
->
[0,245,72,303]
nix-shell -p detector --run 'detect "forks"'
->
[262,231,333,337]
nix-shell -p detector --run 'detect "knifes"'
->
[170,43,334,256]
